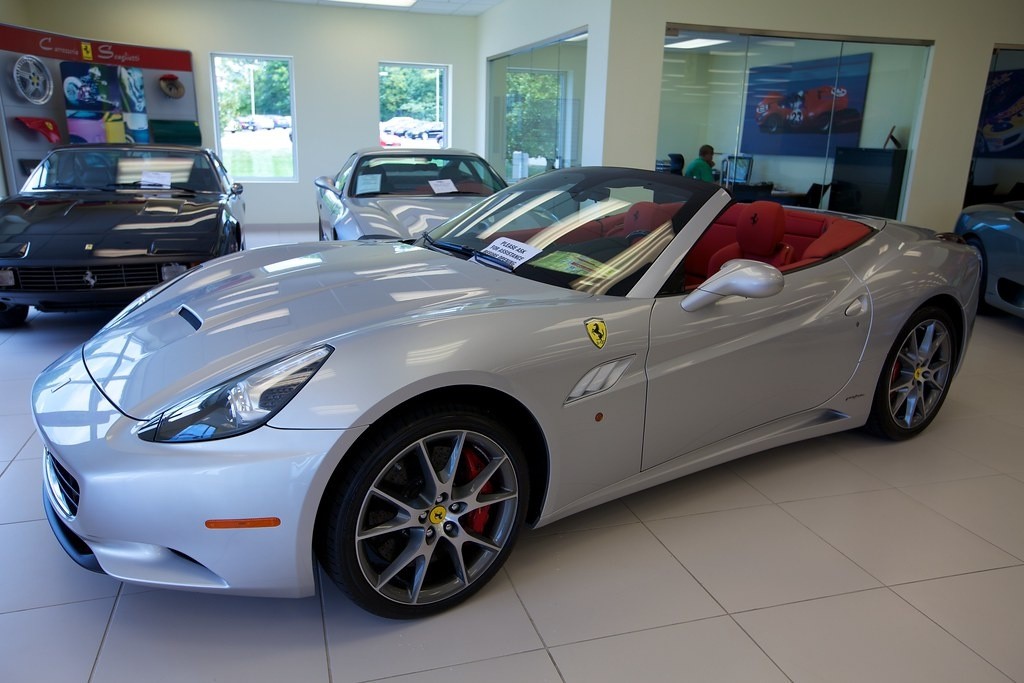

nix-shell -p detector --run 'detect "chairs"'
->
[171,171,204,188]
[783,182,832,209]
[612,201,669,246]
[439,166,468,183]
[707,200,796,284]
[667,153,685,176]
[77,166,112,184]
[354,164,395,193]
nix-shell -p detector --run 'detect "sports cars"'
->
[25,168,986,621]
[315,147,507,249]
[755,86,850,134]
[0,148,247,336]
[952,196,1024,321]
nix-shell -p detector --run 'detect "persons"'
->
[684,144,733,193]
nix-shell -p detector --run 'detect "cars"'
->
[382,116,443,145]
[231,112,291,141]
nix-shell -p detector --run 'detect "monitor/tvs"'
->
[723,154,754,183]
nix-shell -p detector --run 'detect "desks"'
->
[717,181,774,194]
